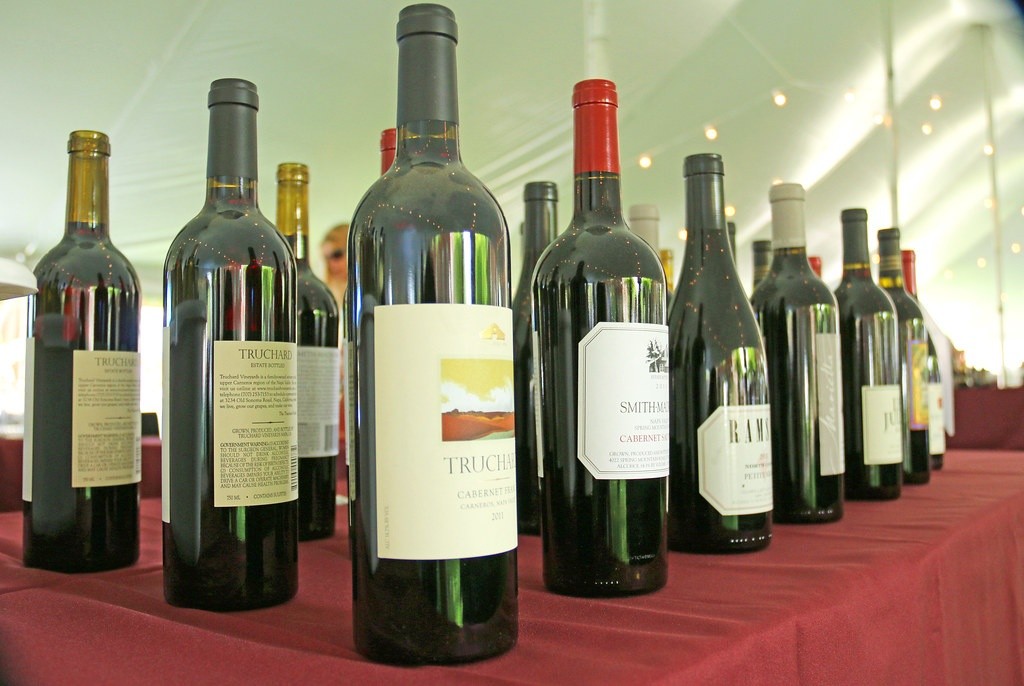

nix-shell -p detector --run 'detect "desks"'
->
[0,383,1024,686]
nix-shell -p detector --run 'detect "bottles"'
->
[162,0,945,667]
[21,131,142,574]
